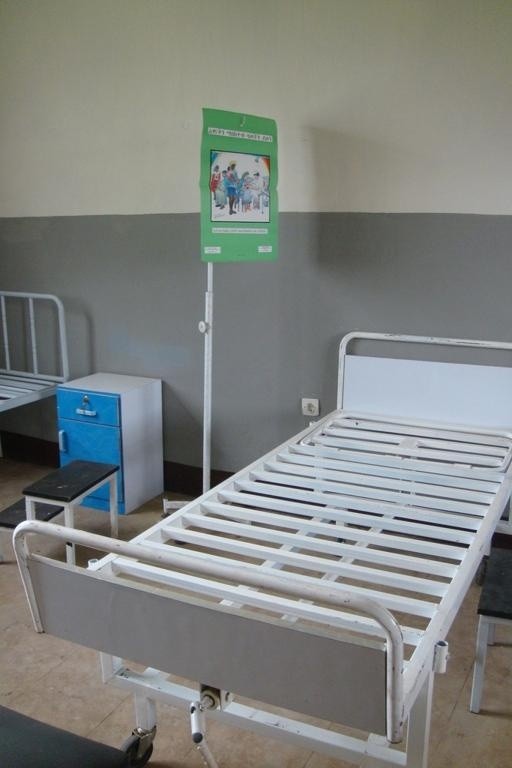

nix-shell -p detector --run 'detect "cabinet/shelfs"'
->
[0,460,120,565]
[55,372,164,515]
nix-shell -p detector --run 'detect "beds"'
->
[11,329,512,768]
[0,290,71,416]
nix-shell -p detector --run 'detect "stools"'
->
[468,529,512,715]
